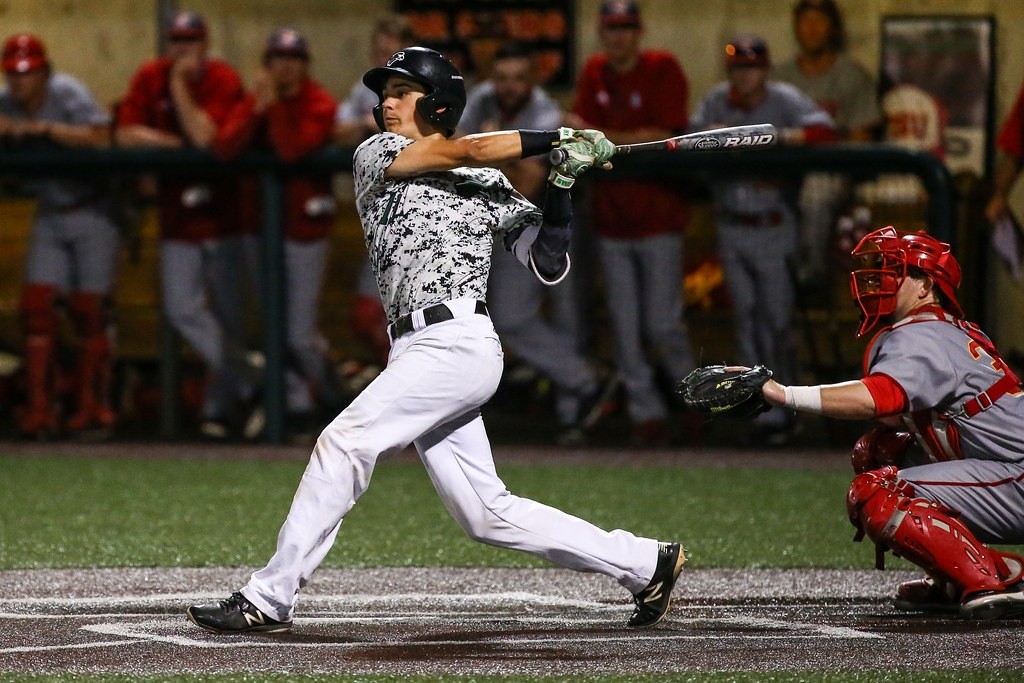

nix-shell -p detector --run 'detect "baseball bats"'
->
[547,121,780,168]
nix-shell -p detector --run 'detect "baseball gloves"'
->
[674,346,775,428]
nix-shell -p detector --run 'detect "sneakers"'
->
[960,577,1024,617]
[892,576,961,610]
[626,540,689,628]
[186,589,293,634]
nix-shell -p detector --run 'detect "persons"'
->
[341,13,618,448]
[207,28,339,438]
[691,33,835,444]
[182,45,686,632]
[679,224,1024,620]
[0,33,119,435]
[984,82,1024,220]
[766,0,881,322]
[112,8,267,440]
[822,83,1024,353]
[563,0,697,441]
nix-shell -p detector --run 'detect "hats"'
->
[267,28,310,60]
[599,0,642,28]
[164,12,207,41]
[724,34,771,66]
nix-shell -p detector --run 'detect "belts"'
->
[390,301,487,341]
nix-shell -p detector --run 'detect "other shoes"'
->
[14,355,821,452]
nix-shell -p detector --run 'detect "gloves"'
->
[548,138,597,189]
[557,126,617,170]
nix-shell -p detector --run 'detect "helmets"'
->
[363,47,466,139]
[792,0,840,34]
[0,33,52,80]
[876,230,964,319]
